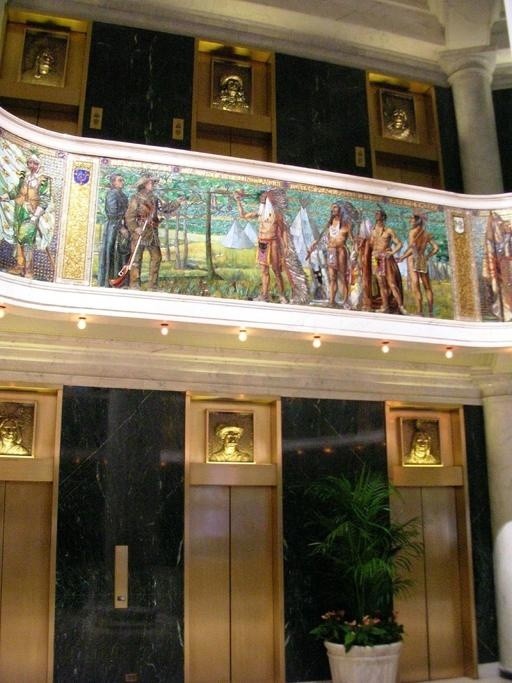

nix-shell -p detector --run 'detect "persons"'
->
[123,174,186,292]
[311,201,439,317]
[405,431,438,465]
[385,106,416,144]
[209,423,252,462]
[23,46,61,87]
[1,154,52,279]
[235,189,289,303]
[1,414,31,456]
[214,70,249,114]
[97,174,130,288]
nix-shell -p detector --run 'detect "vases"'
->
[323,637,403,683]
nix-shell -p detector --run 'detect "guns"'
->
[109,220,148,287]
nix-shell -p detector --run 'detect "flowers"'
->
[283,462,433,651]
